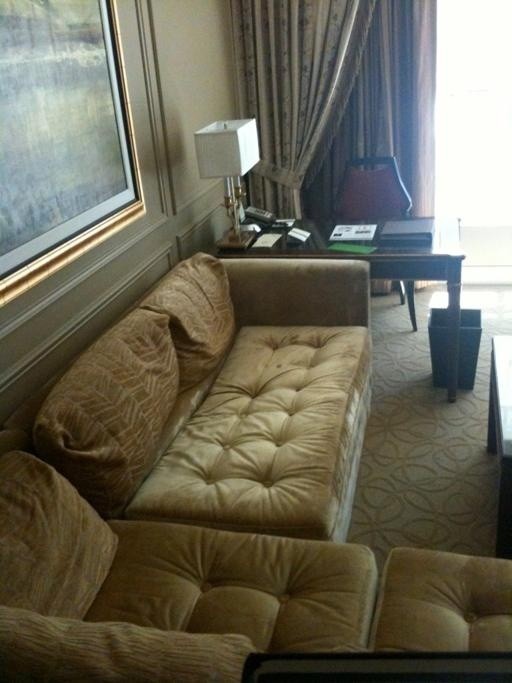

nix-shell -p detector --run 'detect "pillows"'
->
[142,254,239,396]
[29,309,183,518]
[0,452,118,620]
[1,603,267,683]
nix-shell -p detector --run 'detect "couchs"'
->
[1,451,378,681]
[364,545,512,652]
[3,253,374,542]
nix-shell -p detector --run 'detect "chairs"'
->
[334,156,418,332]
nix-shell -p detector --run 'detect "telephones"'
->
[245,206,276,223]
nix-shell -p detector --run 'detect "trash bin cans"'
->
[428,308,482,390]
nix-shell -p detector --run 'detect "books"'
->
[329,224,377,240]
[379,219,435,240]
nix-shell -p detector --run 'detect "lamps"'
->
[193,118,259,248]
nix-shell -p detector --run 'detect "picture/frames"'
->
[0,0,148,307]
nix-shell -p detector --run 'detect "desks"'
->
[205,216,466,403]
